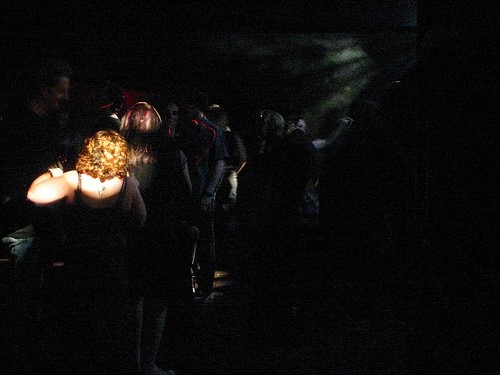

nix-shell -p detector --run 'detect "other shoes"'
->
[214,270,231,279]
[192,291,206,299]
[140,365,176,375]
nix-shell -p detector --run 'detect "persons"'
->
[0,63,356,375]
[26,130,148,374]
